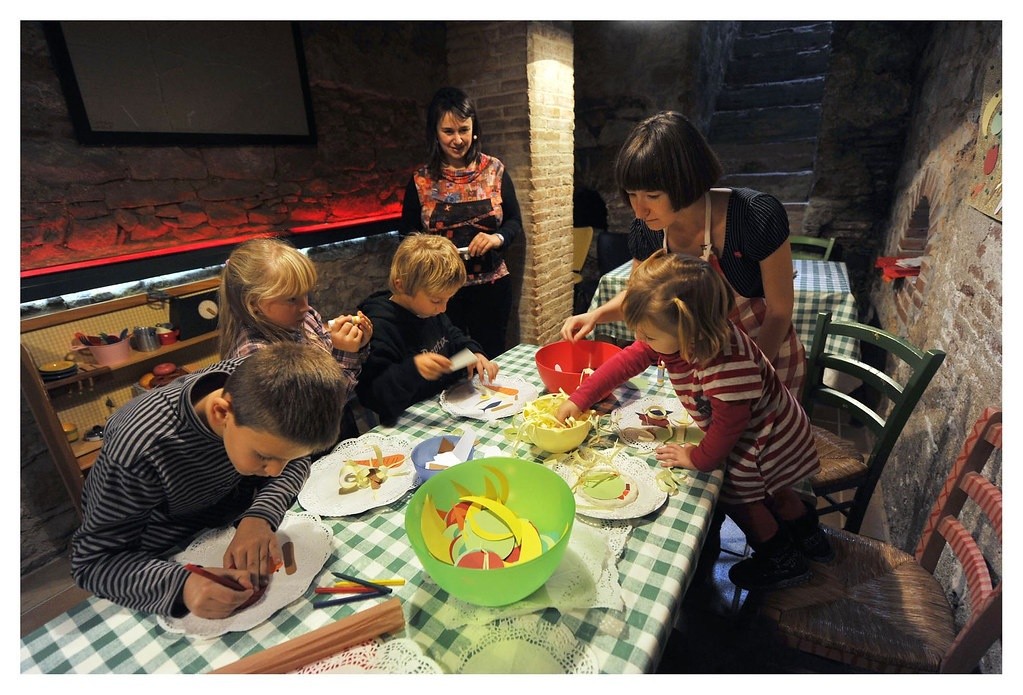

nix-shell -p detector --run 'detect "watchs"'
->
[496,233,505,242]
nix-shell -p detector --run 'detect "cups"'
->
[133,327,160,352]
[70,334,134,364]
[158,329,179,345]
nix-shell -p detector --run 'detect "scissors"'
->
[146,287,181,302]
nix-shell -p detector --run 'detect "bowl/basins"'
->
[405,457,575,607]
[535,340,624,399]
[411,435,473,481]
[523,397,592,453]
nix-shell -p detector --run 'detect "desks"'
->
[585,259,864,395]
[20,343,727,673]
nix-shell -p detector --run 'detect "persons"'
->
[216,239,374,463]
[402,87,522,361]
[67,342,347,619]
[560,110,807,407]
[353,232,500,425]
[557,249,838,591]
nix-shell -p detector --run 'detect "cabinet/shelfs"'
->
[20,275,222,521]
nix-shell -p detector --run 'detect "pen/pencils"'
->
[313,592,383,609]
[315,587,377,593]
[184,562,243,590]
[331,571,392,594]
[334,578,404,587]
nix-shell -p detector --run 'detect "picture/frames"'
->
[42,22,319,147]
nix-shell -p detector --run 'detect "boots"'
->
[728,530,815,590]
[776,500,835,563]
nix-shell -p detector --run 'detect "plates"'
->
[38,361,78,380]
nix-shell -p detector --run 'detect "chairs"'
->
[571,226,593,286]
[788,234,836,261]
[721,310,947,560]
[737,404,1002,674]
